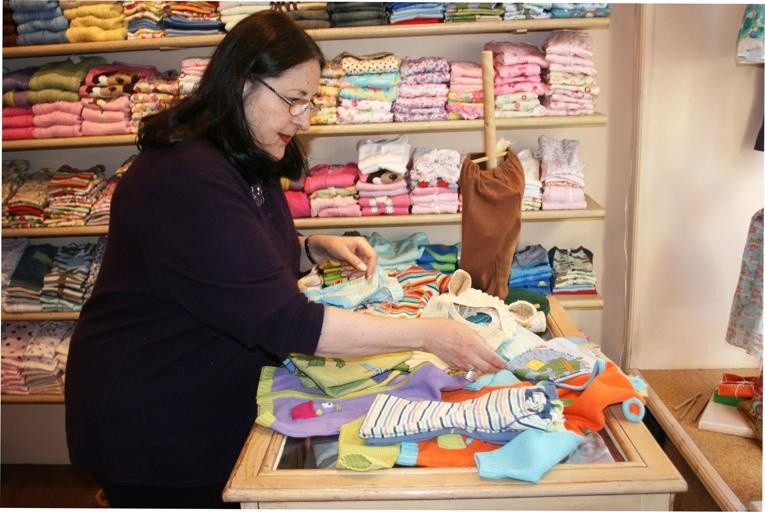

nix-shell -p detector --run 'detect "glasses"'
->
[252,75,323,119]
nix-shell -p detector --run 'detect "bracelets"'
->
[304,233,317,265]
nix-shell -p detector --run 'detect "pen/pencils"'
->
[674,391,713,422]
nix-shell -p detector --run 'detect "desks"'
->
[218,291,690,511]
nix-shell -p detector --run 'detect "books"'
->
[697,380,758,441]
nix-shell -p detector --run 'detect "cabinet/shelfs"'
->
[2,16,611,467]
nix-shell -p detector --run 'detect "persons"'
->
[65,13,506,507]
